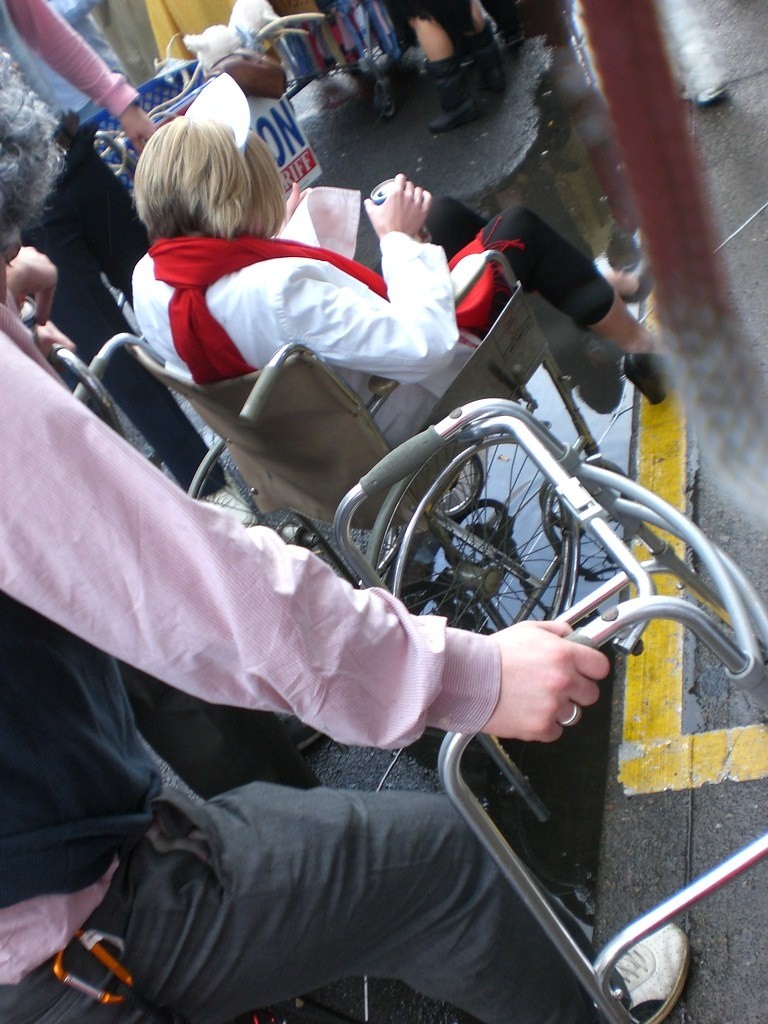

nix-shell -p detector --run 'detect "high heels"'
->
[624,352,674,405]
[621,269,651,304]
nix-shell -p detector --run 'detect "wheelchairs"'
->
[74,248,626,651]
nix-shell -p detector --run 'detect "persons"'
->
[481,72,654,417]
[125,73,685,452]
[2,247,687,1024]
[389,0,586,134]
[0,53,66,306]
[1,2,259,526]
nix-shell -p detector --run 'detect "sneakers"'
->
[600,923,688,1024]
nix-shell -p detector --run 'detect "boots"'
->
[426,57,479,132]
[467,18,506,94]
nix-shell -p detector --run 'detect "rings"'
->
[561,703,579,726]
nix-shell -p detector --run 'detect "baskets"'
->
[66,61,218,191]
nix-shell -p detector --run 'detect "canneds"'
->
[20,295,41,350]
[370,178,433,244]
